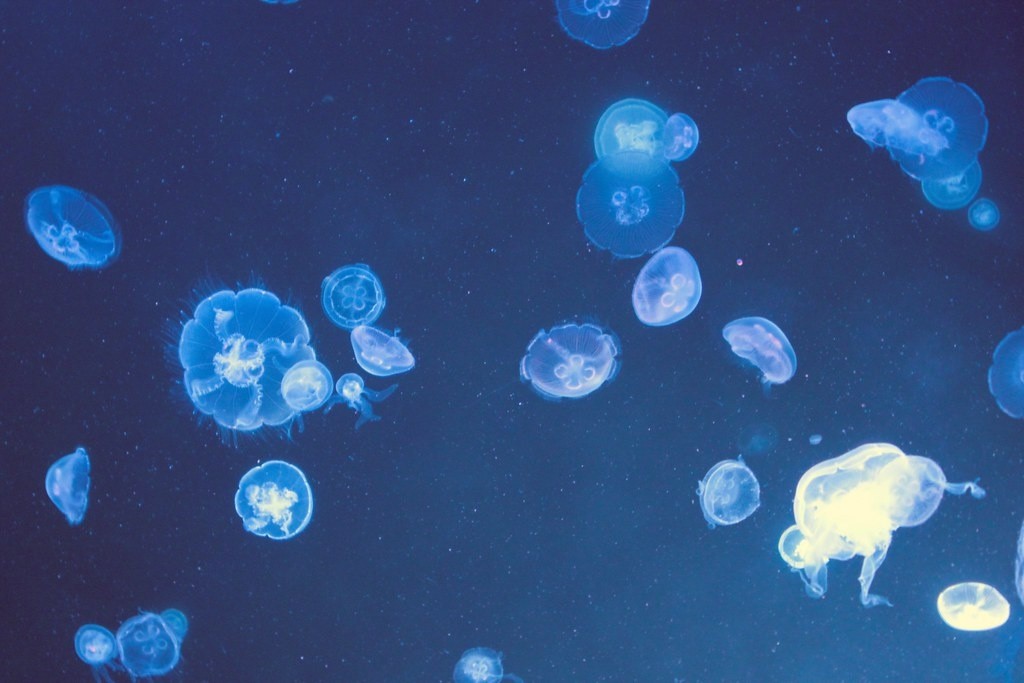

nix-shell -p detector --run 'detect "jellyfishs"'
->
[0,1,1024,682]
